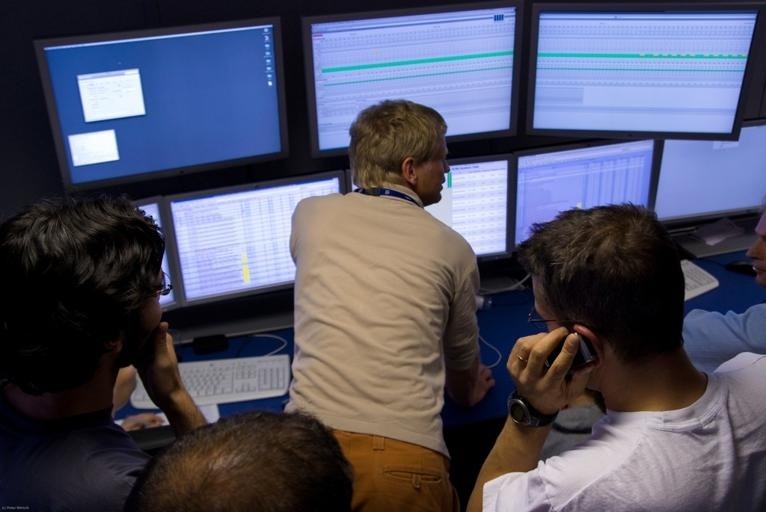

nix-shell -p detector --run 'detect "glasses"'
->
[526,304,587,329]
[143,273,173,302]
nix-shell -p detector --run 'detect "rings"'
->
[513,353,529,364]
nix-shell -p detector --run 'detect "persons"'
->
[681,209,766,373]
[464,203,766,511]
[283,99,496,512]
[118,409,353,512]
[0,190,209,511]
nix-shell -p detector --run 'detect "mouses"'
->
[726,260,757,277]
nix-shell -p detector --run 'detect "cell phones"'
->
[543,331,598,384]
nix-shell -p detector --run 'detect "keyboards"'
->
[129,353,291,411]
[680,258,720,302]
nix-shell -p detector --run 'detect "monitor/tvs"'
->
[513,138,659,259]
[525,0,766,141]
[346,154,513,264]
[652,119,766,236]
[301,0,525,160]
[32,16,291,195]
[165,171,347,309]
[126,196,182,313]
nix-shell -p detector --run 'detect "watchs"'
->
[507,389,560,428]
